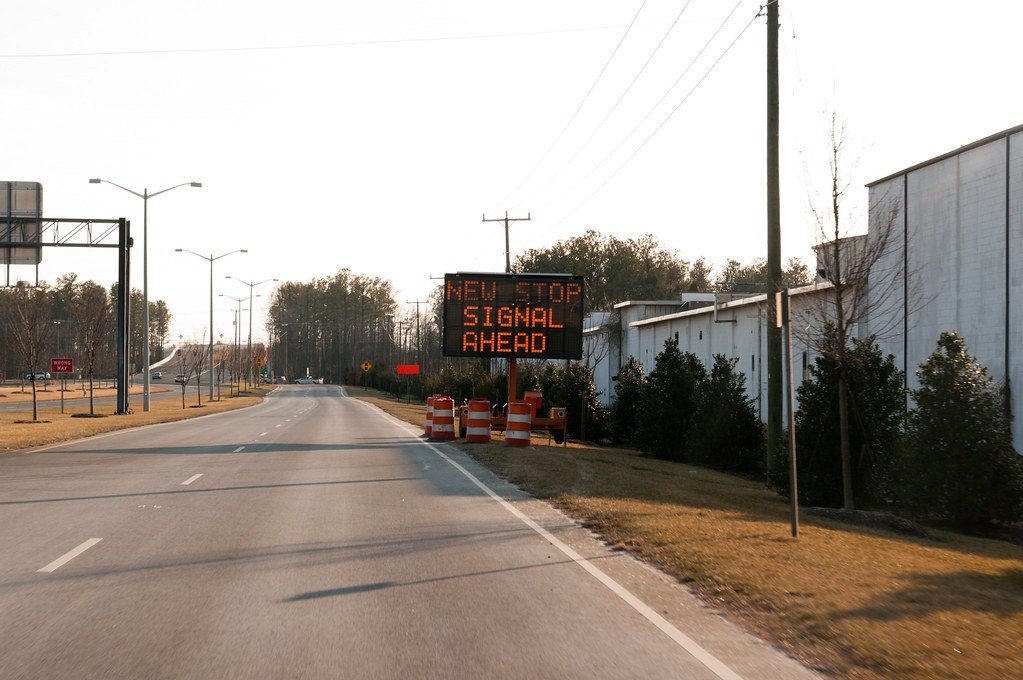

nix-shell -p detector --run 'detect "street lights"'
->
[223,275,279,388]
[174,248,248,402]
[53,321,63,379]
[88,176,204,414]
[217,294,262,386]
[226,309,251,380]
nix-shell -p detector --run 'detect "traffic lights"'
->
[177,349,182,356]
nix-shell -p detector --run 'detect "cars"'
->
[152,372,162,379]
[175,374,187,382]
[313,377,332,384]
[26,371,51,380]
[294,376,313,384]
[264,375,286,384]
